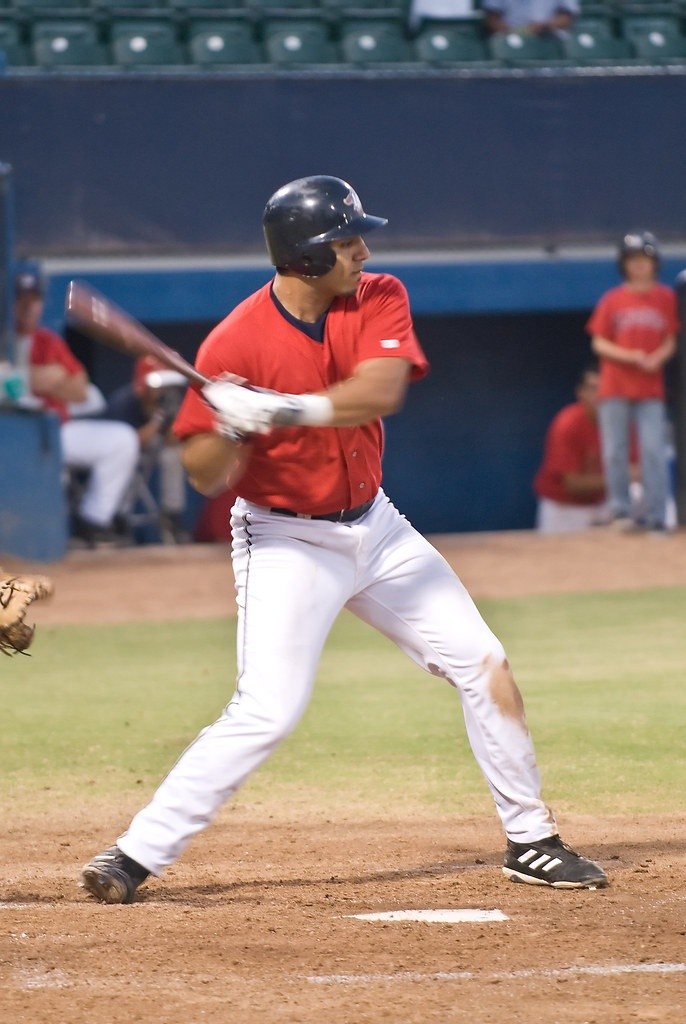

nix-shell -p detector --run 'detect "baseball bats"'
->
[64,277,258,443]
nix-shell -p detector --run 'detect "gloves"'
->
[200,372,305,443]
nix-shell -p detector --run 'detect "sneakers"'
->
[502,835,608,890]
[79,845,149,906]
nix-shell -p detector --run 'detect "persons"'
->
[1,264,234,548]
[83,173,607,903]
[534,228,686,536]
[409,0,581,36]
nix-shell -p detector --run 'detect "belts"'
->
[271,497,376,524]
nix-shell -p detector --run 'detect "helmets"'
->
[262,175,388,278]
[617,231,661,277]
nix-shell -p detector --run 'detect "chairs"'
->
[0,0,686,65]
[19,382,174,550]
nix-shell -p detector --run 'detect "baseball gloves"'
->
[0,561,56,653]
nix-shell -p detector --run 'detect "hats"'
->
[14,272,41,301]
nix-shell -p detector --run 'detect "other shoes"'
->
[68,518,119,551]
